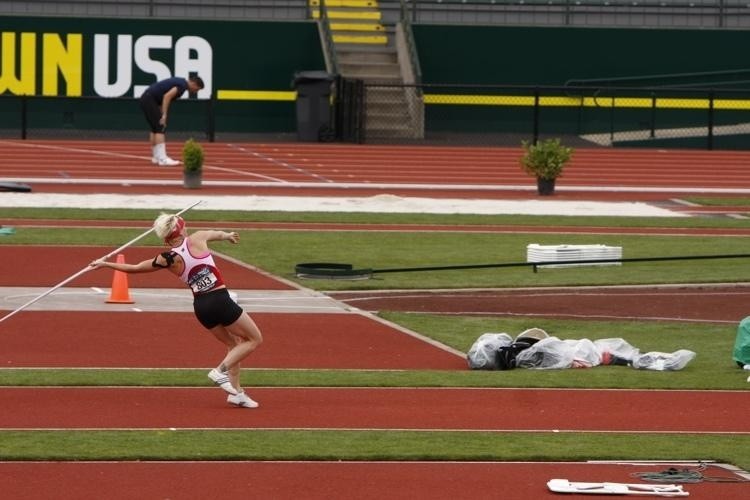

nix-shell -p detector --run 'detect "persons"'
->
[88,213,264,408]
[140,76,204,166]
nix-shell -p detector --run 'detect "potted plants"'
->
[518,137,572,195]
[182,139,203,188]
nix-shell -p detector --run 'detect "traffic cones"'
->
[105,254,136,305]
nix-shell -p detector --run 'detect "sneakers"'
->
[158,158,180,166]
[226,393,259,409]
[151,157,159,165]
[207,368,238,395]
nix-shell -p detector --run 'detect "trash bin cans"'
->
[289,70,341,143]
